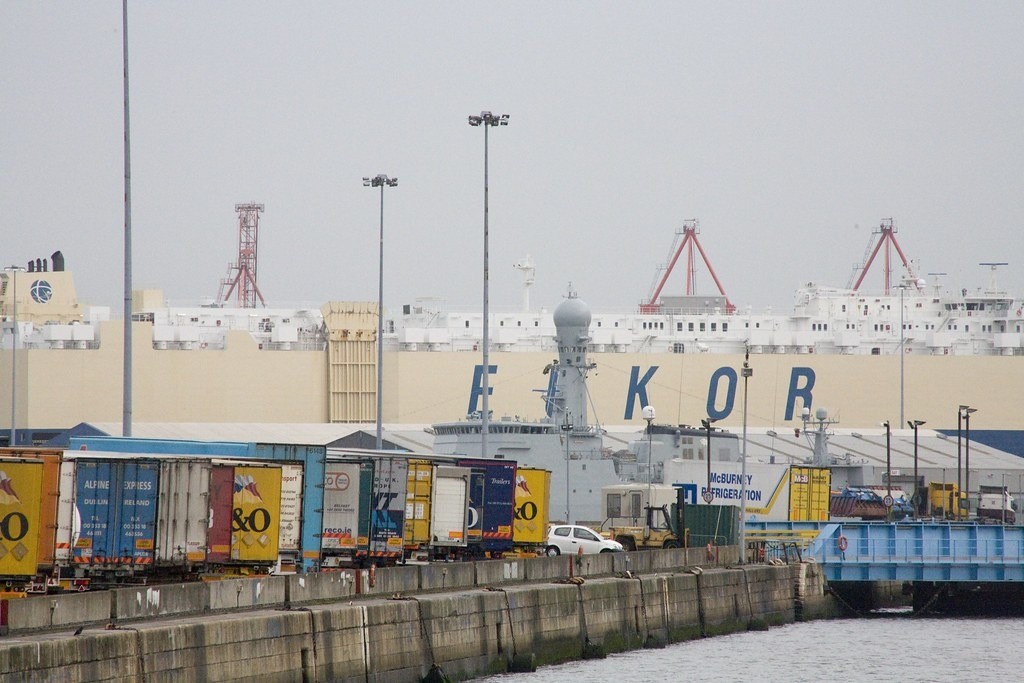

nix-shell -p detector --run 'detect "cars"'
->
[977,485,1015,523]
[545,526,623,557]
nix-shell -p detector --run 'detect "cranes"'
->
[235,203,265,308]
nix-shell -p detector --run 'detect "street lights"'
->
[1002,472,1011,525]
[469,110,512,457]
[736,368,753,564]
[963,409,978,520]
[907,420,926,522]
[958,405,970,521]
[701,418,717,504]
[362,173,399,450]
[883,423,891,524]
[561,423,574,525]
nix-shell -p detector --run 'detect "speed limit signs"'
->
[703,492,714,503]
[884,495,893,507]
